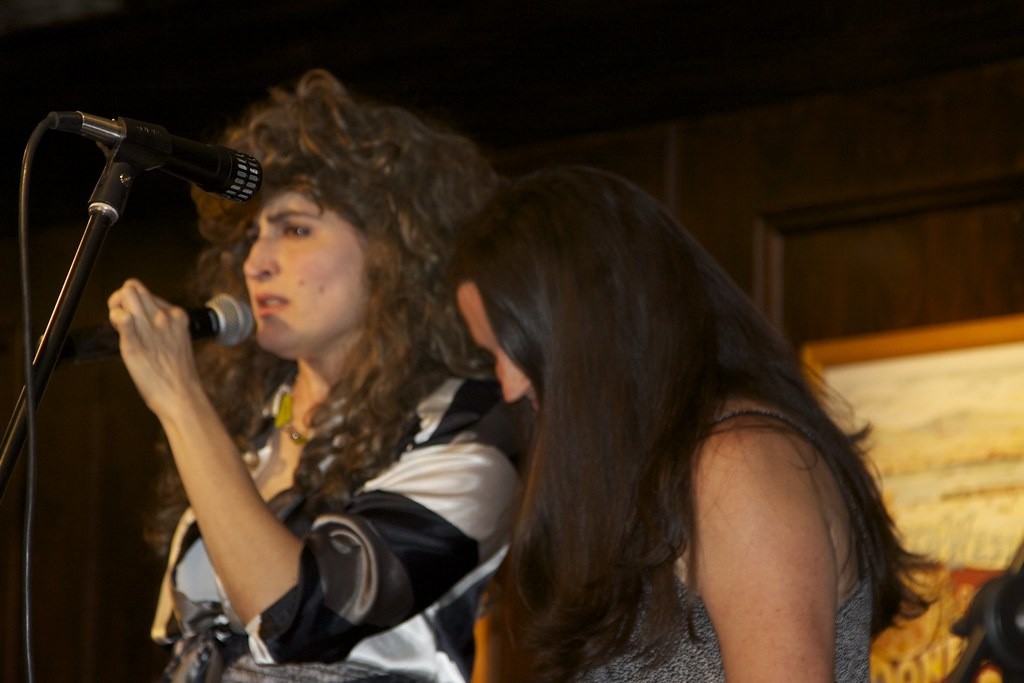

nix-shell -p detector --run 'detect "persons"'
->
[108,66,937,683]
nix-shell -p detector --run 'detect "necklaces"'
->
[285,422,305,443]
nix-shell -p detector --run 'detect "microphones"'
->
[48,111,263,202]
[56,293,252,366]
